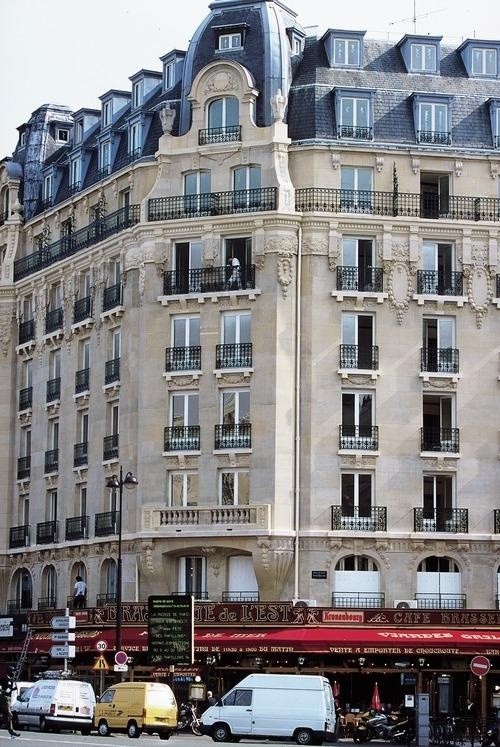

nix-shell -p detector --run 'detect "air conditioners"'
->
[393,599,417,610]
[292,600,316,608]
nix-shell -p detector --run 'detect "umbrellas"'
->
[371,682,382,713]
[332,680,338,706]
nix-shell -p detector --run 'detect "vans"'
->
[10,678,97,737]
[197,673,338,745]
[93,681,179,739]
[7,681,35,707]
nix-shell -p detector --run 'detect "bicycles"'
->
[428,713,480,744]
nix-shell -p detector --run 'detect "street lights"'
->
[105,463,138,683]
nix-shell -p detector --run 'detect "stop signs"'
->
[470,655,491,675]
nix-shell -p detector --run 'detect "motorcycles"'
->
[177,703,203,736]
[483,710,499,746]
[353,710,415,743]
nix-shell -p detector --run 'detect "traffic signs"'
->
[48,644,77,657]
[50,615,76,629]
[96,639,108,652]
[49,631,75,641]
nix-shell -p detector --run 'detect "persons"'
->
[463,698,477,737]
[207,690,218,706]
[72,576,87,609]
[226,257,244,290]
[0,666,20,737]
